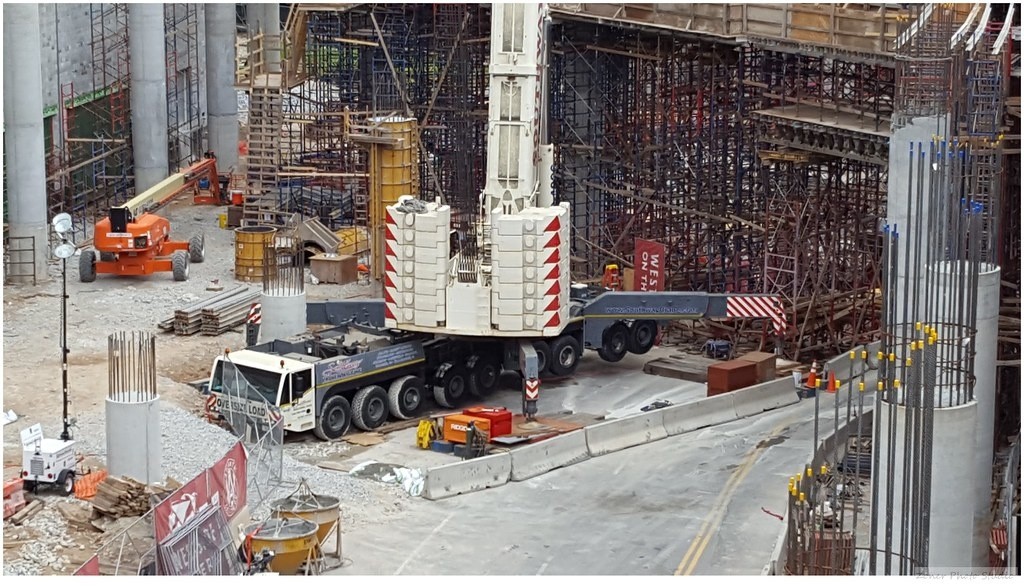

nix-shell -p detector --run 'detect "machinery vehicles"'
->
[200,2,790,440]
[20,211,79,499]
[76,155,230,278]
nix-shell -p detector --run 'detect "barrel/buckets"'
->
[232,192,242,205]
[234,225,277,283]
[219,213,228,229]
